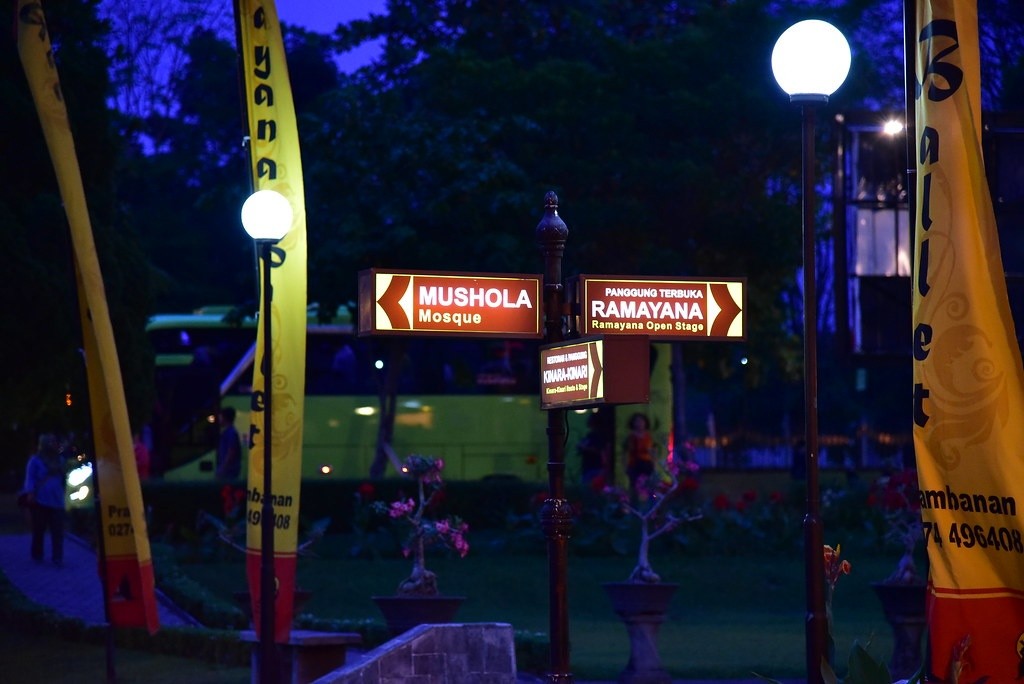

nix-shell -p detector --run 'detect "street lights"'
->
[769,17,851,684]
[241,190,296,684]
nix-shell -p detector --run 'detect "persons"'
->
[622,414,660,504]
[575,412,610,515]
[129,423,150,519]
[214,407,242,541]
[20,431,74,567]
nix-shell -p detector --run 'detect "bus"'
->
[65,304,671,528]
